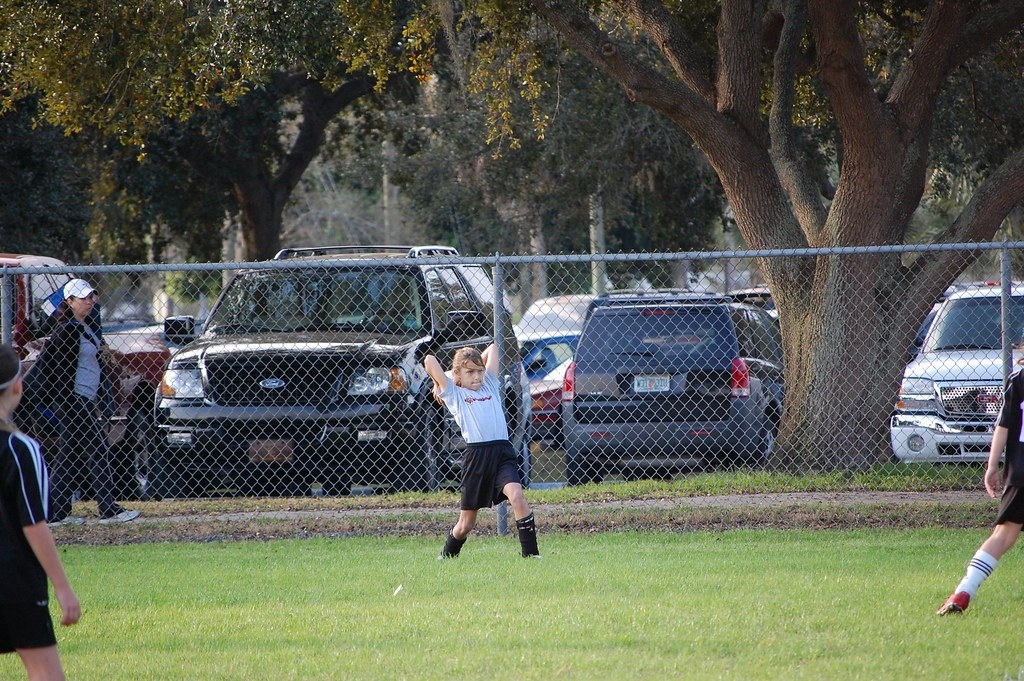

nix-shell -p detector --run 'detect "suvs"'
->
[143,243,536,499]
[0,253,171,486]
[891,280,1024,466]
[562,291,787,486]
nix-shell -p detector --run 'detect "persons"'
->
[936,343,1024,617]
[46,278,141,528]
[424,344,539,560]
[0,343,81,681]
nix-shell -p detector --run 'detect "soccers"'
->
[438,370,454,406]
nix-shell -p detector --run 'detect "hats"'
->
[64,279,98,300]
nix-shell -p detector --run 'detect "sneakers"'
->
[936,592,971,615]
[46,516,84,528]
[99,510,141,526]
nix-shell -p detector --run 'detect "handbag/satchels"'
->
[97,375,116,420]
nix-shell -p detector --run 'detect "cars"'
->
[514,292,610,443]
